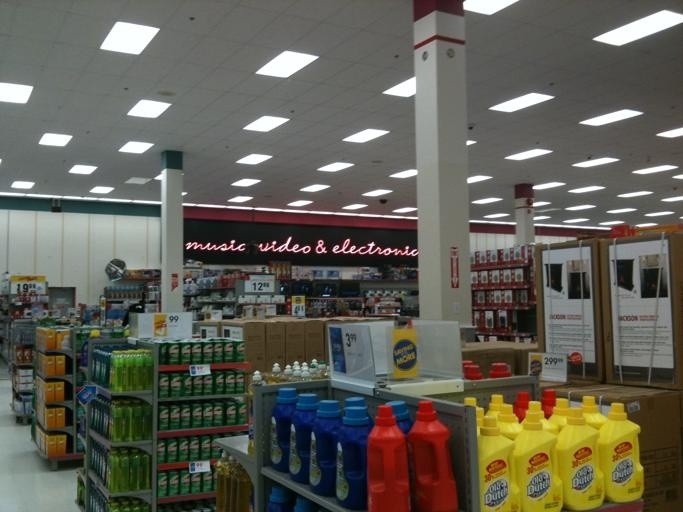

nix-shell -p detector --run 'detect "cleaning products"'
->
[268,361,646,511]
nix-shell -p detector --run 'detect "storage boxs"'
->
[545,383,681,510]
[598,232,683,390]
[539,239,605,382]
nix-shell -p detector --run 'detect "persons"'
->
[118,292,145,327]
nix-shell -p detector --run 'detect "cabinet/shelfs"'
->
[101,292,147,327]
[84,337,154,512]
[249,377,479,512]
[34,323,127,469]
[1,290,99,427]
[182,266,419,322]
[471,246,537,345]
[154,339,253,512]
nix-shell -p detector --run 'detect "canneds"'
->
[159,337,248,497]
